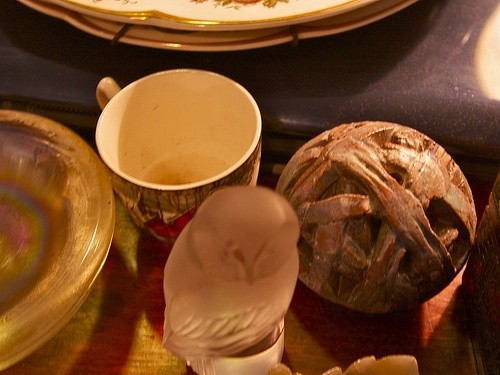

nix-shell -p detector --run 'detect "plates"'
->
[20,0,419,52]
[48,0,376,32]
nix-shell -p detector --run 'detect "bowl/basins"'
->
[0,109,115,372]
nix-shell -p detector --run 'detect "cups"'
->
[95,69,263,242]
